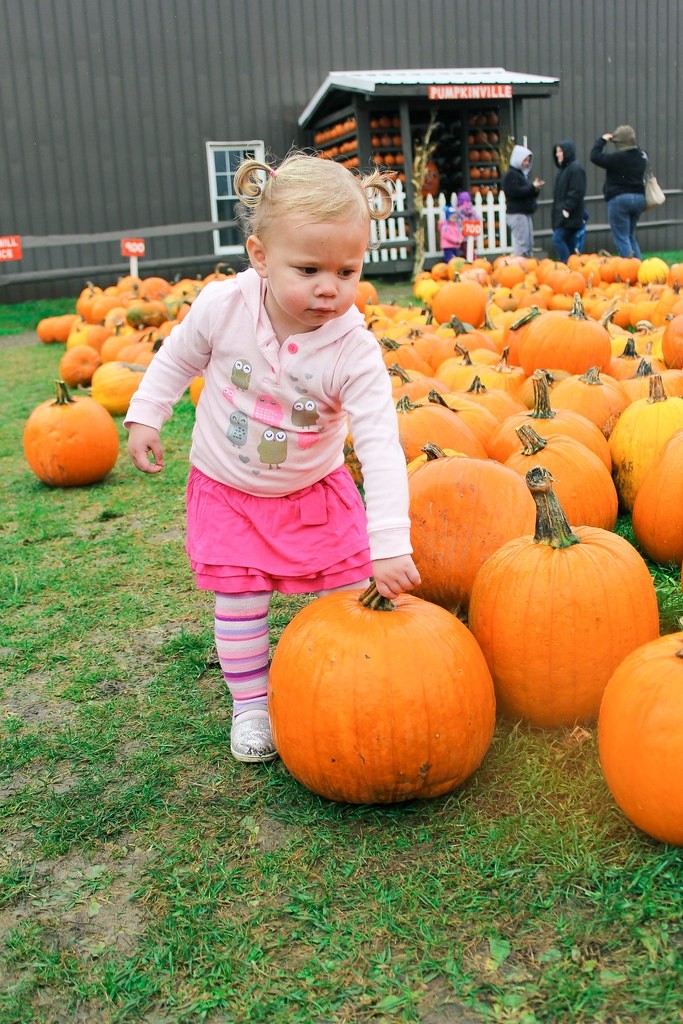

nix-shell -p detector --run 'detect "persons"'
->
[590,125,650,261]
[438,206,464,263]
[122,146,422,763]
[503,145,546,258]
[550,140,588,265]
[574,206,590,255]
[455,192,484,262]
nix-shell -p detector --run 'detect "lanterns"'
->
[420,158,439,196]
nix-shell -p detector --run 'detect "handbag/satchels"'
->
[642,167,666,209]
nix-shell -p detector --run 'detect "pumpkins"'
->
[21,253,683,844]
[314,112,503,249]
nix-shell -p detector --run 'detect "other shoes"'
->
[230,701,277,762]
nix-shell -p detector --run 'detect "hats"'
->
[456,191,472,205]
[442,205,456,220]
